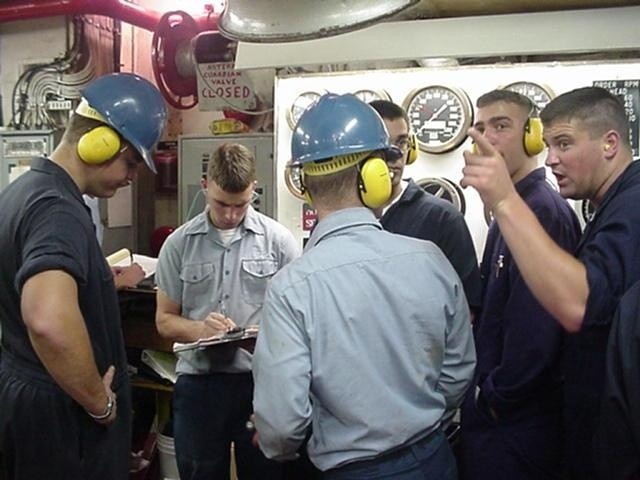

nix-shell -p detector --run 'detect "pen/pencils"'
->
[496,255,504,278]
[130,249,133,266]
[218,301,226,319]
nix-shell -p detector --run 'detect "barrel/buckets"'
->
[154,433,179,478]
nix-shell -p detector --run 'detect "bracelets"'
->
[87,393,113,420]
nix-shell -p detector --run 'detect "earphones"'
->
[603,143,611,152]
[204,188,208,192]
[252,190,255,194]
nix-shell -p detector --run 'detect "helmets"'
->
[76,72,169,175]
[284,92,402,177]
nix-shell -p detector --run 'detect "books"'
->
[171,324,258,353]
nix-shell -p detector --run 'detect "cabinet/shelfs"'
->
[177,133,273,228]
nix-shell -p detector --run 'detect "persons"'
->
[460,86,639,479]
[251,91,480,480]
[108,260,157,474]
[364,99,484,324]
[0,71,168,480]
[454,88,582,480]
[154,141,302,480]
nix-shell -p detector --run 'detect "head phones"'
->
[76,124,122,164]
[407,133,419,164]
[473,94,543,156]
[301,155,393,210]
